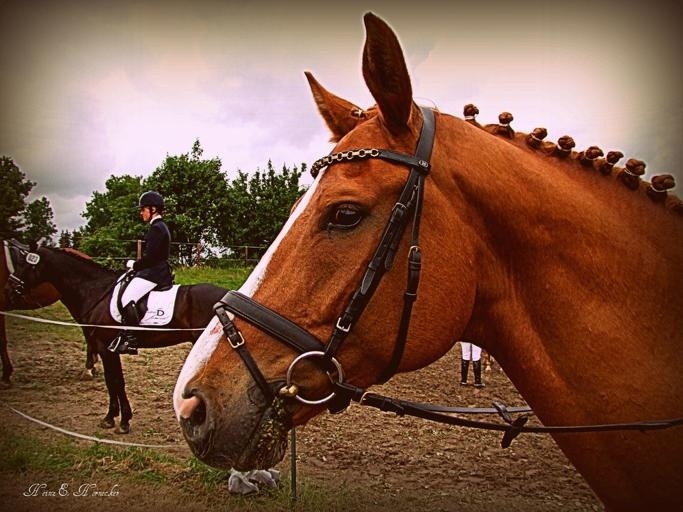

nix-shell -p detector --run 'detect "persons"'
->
[111,191,171,355]
[460,342,486,387]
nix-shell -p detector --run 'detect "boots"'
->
[459,359,470,386]
[112,300,139,355]
[472,359,484,388]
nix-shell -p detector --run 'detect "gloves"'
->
[125,259,136,273]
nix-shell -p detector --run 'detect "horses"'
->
[172,10,682,512]
[4,240,231,435]
[0,238,101,390]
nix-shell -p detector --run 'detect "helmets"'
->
[135,190,164,208]
